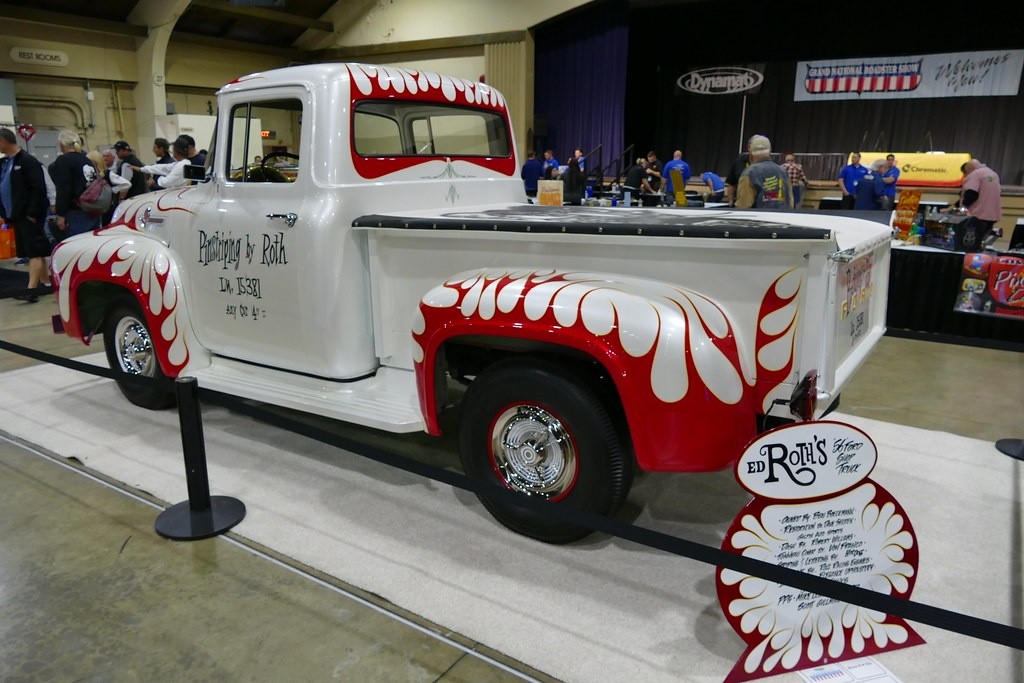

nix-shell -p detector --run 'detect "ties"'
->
[4,158,12,173]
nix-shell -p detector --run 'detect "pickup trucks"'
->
[51,62,893,545]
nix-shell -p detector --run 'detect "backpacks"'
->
[72,162,112,218]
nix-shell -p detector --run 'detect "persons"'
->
[623,157,656,200]
[49,130,208,237]
[521,147,585,206]
[255,156,261,166]
[659,150,691,207]
[640,152,664,194]
[274,151,291,168]
[946,158,1001,253]
[724,134,795,210]
[780,153,808,209]
[838,152,900,211]
[699,171,724,203]
[0,128,57,303]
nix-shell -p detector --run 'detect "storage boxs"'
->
[923,213,981,251]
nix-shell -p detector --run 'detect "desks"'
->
[889,244,1024,344]
[818,197,951,213]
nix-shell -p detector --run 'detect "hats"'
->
[751,135,770,155]
[172,138,189,154]
[173,134,195,146]
[154,137,172,153]
[110,140,131,150]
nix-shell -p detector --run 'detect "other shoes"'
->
[11,284,40,303]
[37,283,54,295]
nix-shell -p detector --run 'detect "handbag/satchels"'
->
[0,225,17,260]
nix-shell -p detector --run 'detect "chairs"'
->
[640,194,662,204]
[687,195,704,206]
[685,190,698,195]
[620,186,637,198]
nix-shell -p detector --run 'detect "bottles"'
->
[585,186,592,199]
[612,195,616,207]
